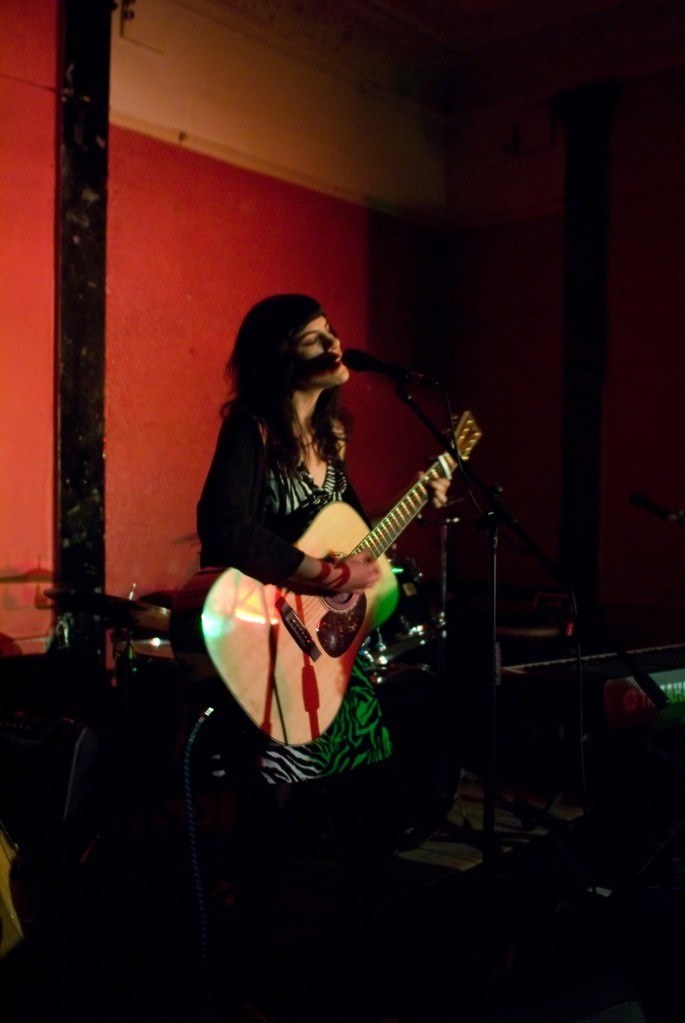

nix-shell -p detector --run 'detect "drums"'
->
[128,637,183,717]
[358,554,442,672]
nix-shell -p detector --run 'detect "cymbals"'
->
[42,586,174,635]
[137,587,179,610]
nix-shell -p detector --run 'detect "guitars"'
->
[168,406,483,756]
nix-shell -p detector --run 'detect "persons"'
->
[196,294,451,810]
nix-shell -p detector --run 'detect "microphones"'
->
[629,492,685,528]
[341,348,430,384]
